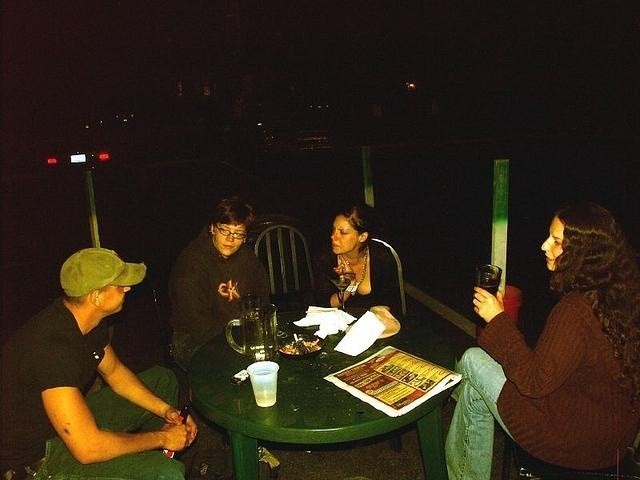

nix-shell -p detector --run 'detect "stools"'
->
[502,435,640,480]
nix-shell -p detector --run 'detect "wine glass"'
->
[326,272,356,315]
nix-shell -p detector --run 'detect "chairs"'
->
[252,220,315,295]
[372,239,407,314]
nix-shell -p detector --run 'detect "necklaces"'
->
[343,248,368,285]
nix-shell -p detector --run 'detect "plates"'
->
[276,334,327,360]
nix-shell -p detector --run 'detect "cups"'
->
[476,264,503,297]
[240,291,261,313]
[225,302,278,364]
[247,361,280,407]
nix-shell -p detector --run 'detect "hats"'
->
[60,247,147,298]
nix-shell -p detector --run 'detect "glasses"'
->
[215,224,248,240]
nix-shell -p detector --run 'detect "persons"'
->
[0,248,198,480]
[171,196,271,373]
[314,200,400,315]
[444,203,640,480]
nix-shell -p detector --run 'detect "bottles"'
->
[161,402,195,460]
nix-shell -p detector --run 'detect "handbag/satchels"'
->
[185,448,273,479]
